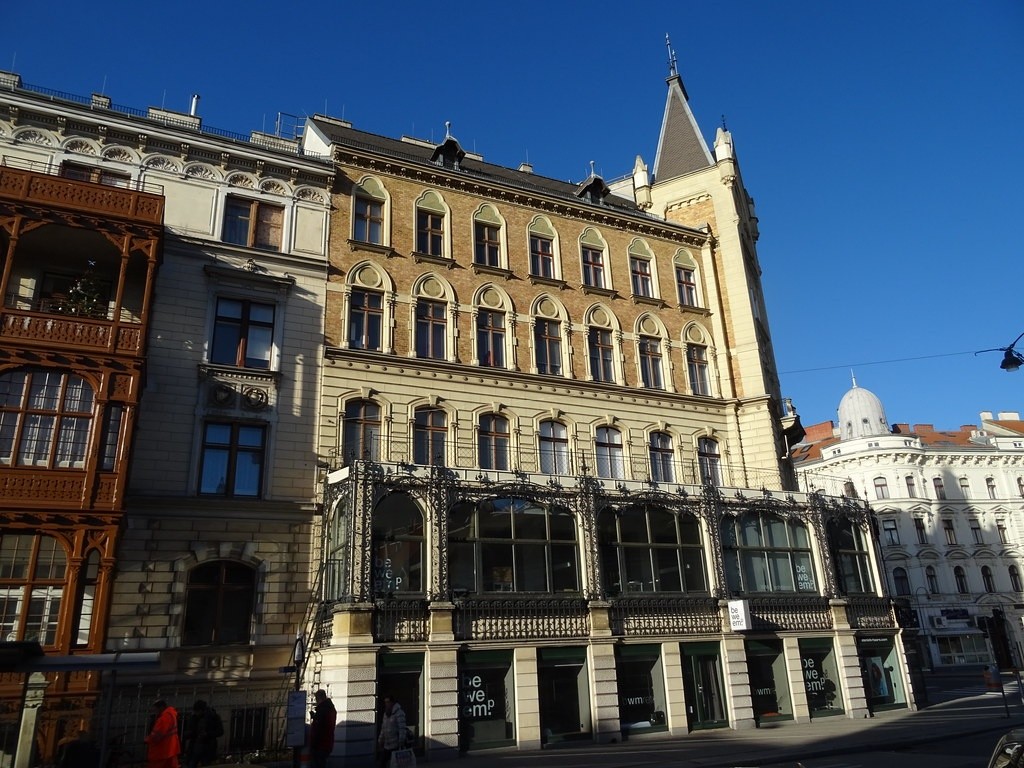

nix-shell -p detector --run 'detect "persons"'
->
[870,663,887,696]
[308,688,336,768]
[179,698,225,768]
[375,695,408,766]
[143,699,181,768]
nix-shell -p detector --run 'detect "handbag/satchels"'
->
[390,749,417,768]
